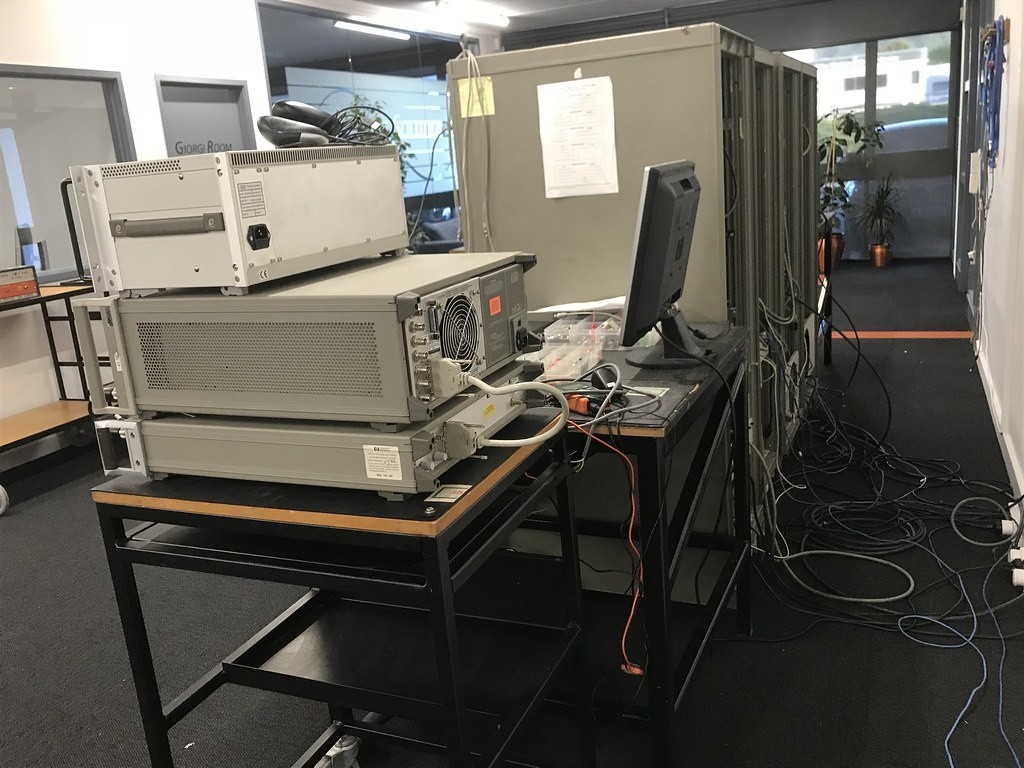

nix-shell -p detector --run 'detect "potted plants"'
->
[847,171,909,268]
[818,109,887,273]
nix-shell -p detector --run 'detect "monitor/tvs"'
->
[618,160,717,368]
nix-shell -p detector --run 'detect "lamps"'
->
[333,20,414,41]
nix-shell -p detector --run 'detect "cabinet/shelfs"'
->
[93,410,597,768]
[0,284,118,516]
[446,23,820,614]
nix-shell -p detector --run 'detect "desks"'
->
[516,323,759,768]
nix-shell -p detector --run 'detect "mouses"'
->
[590,368,623,399]
[256,99,342,148]
